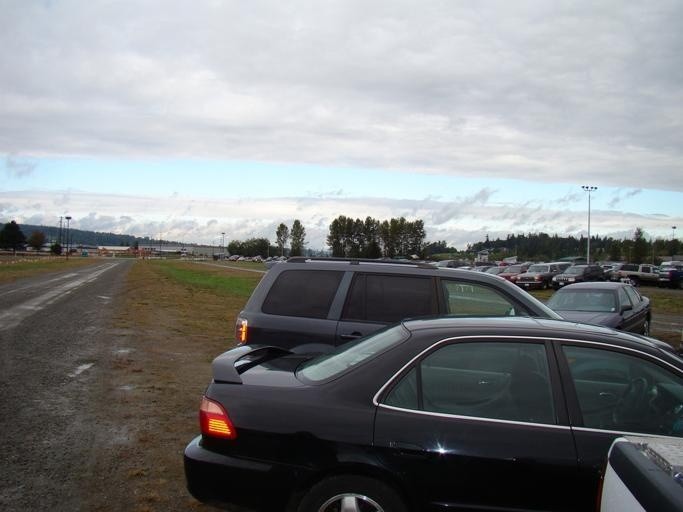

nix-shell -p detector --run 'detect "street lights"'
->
[221,232,225,253]
[580,184,598,264]
[65,216,71,260]
[670,226,676,241]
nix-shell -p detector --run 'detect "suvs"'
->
[230,252,566,361]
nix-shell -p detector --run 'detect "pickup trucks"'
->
[589,427,682,512]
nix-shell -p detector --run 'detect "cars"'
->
[433,255,683,290]
[543,279,655,340]
[179,315,682,512]
[230,253,287,268]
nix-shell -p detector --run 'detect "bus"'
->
[180,251,188,257]
